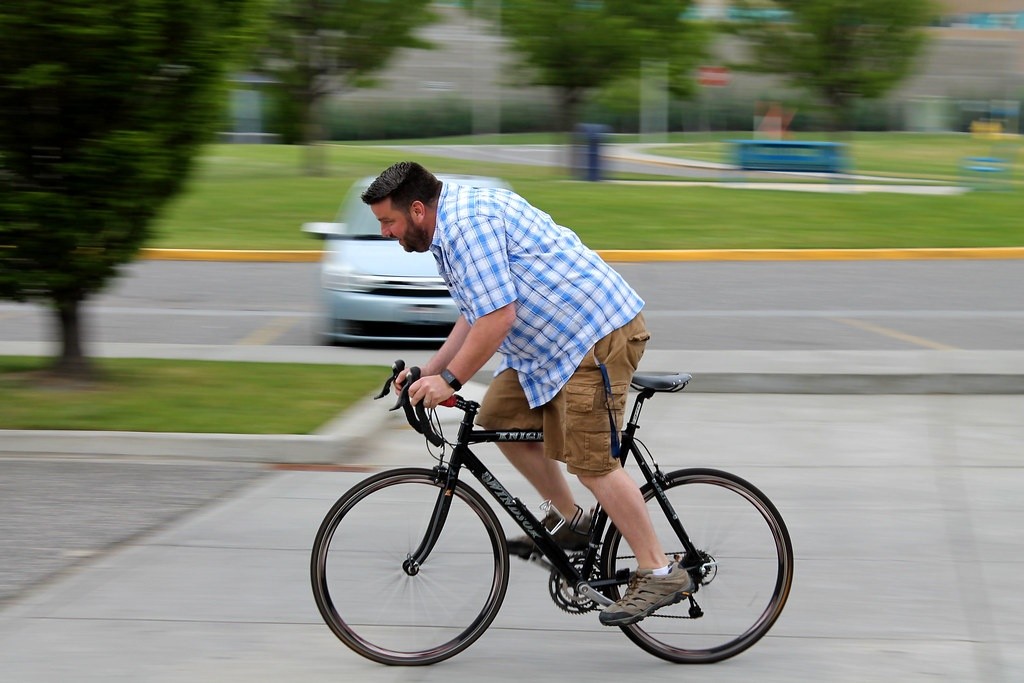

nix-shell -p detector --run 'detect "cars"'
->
[300,174,515,349]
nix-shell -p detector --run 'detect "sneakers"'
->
[599,554,696,626]
[506,506,594,555]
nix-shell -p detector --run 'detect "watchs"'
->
[440,369,462,391]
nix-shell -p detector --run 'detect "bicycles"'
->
[310,357,795,667]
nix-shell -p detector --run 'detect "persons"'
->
[361,161,692,626]
